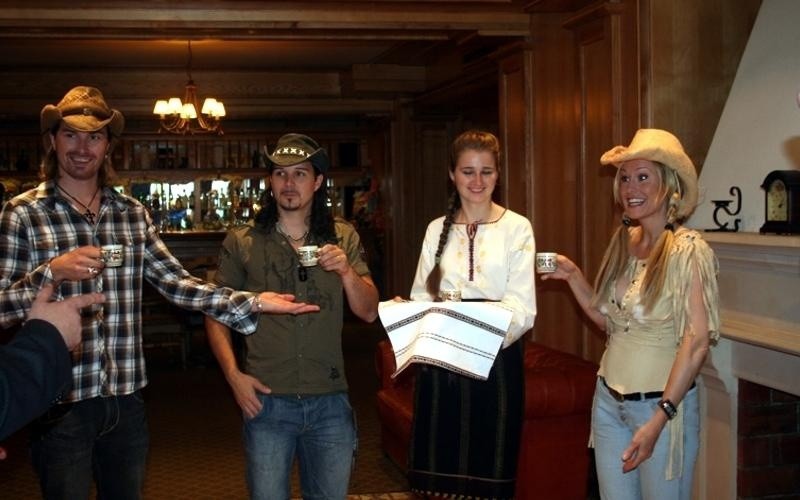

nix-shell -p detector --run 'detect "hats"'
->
[262,131,330,170]
[39,84,126,137]
[599,128,699,218]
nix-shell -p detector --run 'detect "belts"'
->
[600,378,698,403]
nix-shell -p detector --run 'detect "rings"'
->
[87,266,99,276]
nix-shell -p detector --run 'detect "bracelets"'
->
[657,398,679,420]
[255,294,263,314]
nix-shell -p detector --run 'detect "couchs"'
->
[377,339,600,500]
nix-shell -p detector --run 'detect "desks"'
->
[158,225,227,275]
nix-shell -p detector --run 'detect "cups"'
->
[440,290,461,303]
[101,244,123,267]
[298,246,321,266]
[537,253,557,274]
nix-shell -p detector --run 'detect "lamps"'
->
[153,38,226,139]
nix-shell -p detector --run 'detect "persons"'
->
[0,282,107,443]
[540,128,722,499]
[392,129,538,500]
[0,86,320,500]
[204,132,380,500]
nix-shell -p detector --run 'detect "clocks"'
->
[758,170,800,238]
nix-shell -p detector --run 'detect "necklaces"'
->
[278,230,312,282]
[55,181,101,226]
